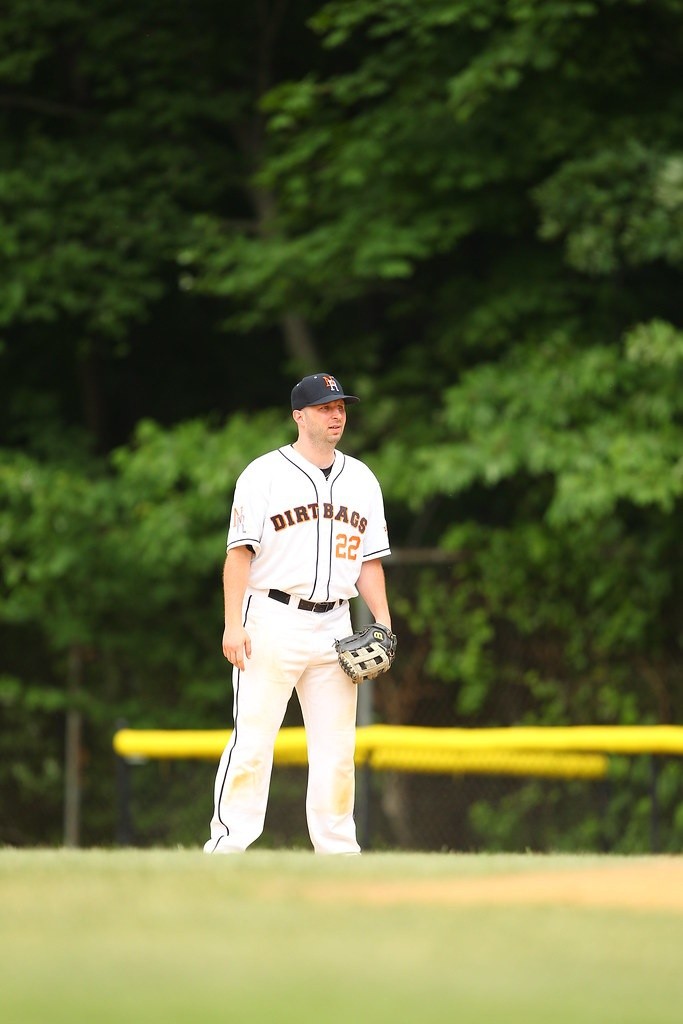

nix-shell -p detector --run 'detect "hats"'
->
[292,373,360,413]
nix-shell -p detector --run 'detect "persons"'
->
[203,372,394,856]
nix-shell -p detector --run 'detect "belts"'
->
[268,589,342,613]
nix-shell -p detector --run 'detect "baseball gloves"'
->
[331,623,397,686]
